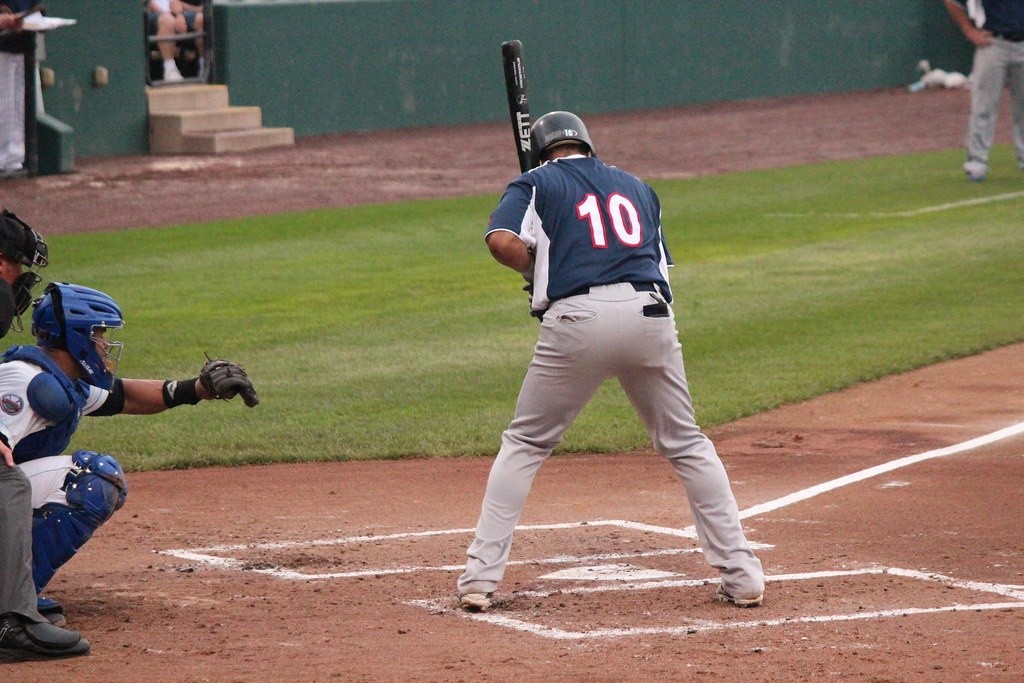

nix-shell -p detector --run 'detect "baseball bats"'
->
[500,39,536,174]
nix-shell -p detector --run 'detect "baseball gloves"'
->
[198,359,260,410]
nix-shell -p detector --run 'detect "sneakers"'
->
[39,612,67,628]
[0,617,90,663]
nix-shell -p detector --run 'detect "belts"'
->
[992,30,1021,43]
[546,281,671,303]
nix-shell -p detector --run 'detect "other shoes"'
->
[165,70,185,82]
[716,586,766,609]
[457,592,491,611]
[962,160,987,181]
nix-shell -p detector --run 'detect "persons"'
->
[146,0,205,83]
[0,0,44,171]
[456,111,767,610]
[0,281,259,625]
[945,0,1024,180]
[0,209,88,660]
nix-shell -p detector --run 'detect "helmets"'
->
[529,111,596,158]
[31,281,124,393]
[0,210,48,266]
